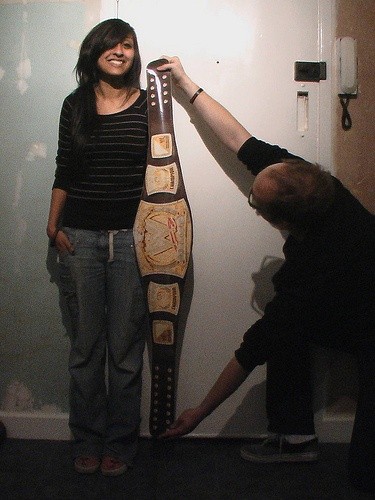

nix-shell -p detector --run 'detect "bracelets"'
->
[50,230,59,247]
[189,87,203,104]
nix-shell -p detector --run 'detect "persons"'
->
[46,19,148,477]
[158,54,375,465]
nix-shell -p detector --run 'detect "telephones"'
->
[336,36,358,98]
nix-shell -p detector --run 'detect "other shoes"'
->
[102,455,125,477]
[75,456,97,474]
[240,431,320,464]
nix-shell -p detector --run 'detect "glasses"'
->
[247,185,266,211]
